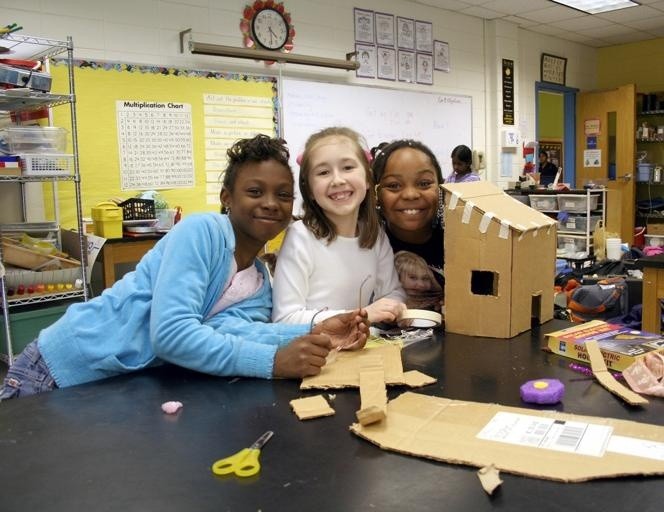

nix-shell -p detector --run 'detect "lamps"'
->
[180,28,361,69]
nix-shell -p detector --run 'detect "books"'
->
[2,221,59,230]
[124,226,157,238]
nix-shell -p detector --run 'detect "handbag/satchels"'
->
[556,250,629,323]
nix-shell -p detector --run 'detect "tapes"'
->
[397,309,442,330]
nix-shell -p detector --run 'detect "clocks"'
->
[252,7,288,50]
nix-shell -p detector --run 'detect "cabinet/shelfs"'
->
[0,34,89,367]
[504,189,606,255]
[635,110,664,218]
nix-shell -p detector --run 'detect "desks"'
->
[1,316,664,511]
[636,253,664,335]
[95,234,164,288]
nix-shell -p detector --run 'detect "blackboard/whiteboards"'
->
[277,76,474,218]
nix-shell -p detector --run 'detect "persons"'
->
[393,250,444,312]
[369,139,445,330]
[538,150,559,185]
[447,145,481,183]
[0,134,371,402]
[271,127,415,329]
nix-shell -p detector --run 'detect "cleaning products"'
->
[174,206,182,225]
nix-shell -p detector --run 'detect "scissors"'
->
[212,430,274,477]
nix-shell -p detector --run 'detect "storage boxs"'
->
[641,163,655,182]
[528,194,600,252]
[0,127,70,154]
[0,306,66,355]
[644,234,664,247]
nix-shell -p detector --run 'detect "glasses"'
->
[311,275,372,369]
